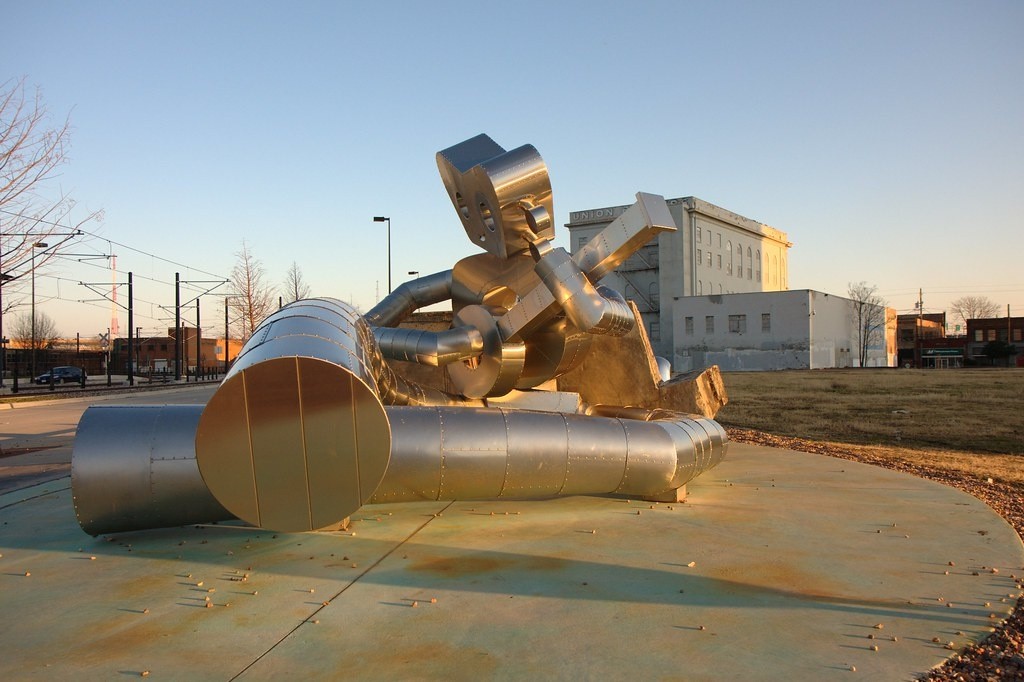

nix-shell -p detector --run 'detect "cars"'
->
[35,367,88,384]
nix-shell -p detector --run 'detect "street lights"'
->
[31,243,48,379]
[373,216,391,297]
[408,271,420,313]
